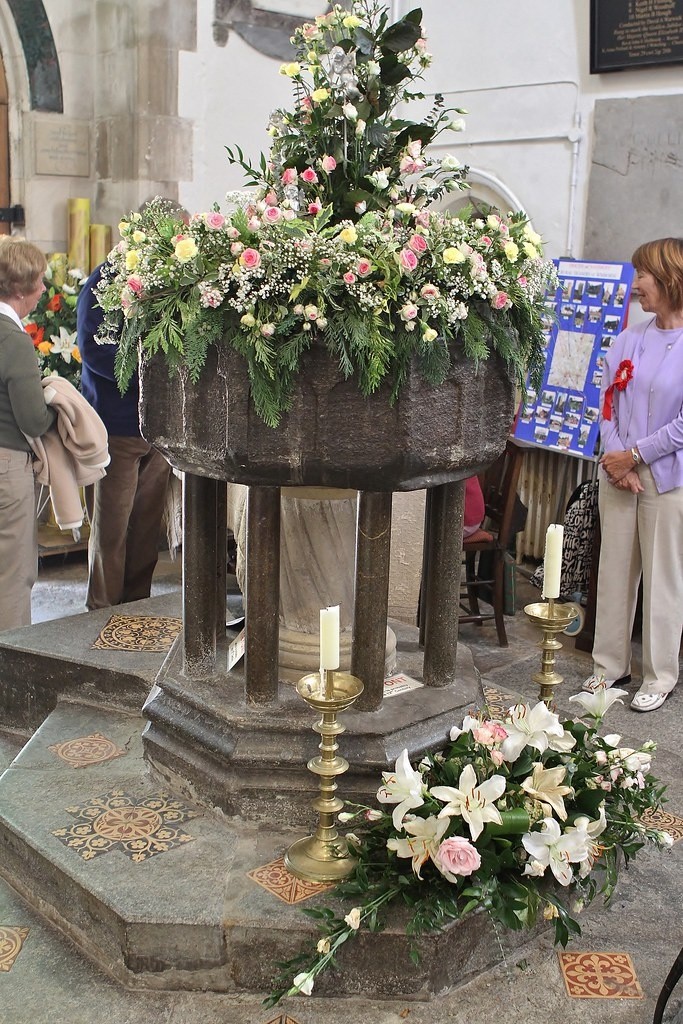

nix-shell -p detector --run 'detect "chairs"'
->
[458,443,525,648]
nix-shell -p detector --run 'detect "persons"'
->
[86,199,190,614]
[0,242,58,635]
[581,238,683,711]
[461,475,485,537]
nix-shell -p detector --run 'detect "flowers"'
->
[89,0,555,430]
[22,258,91,393]
[263,675,677,1009]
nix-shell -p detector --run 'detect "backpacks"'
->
[528,479,600,598]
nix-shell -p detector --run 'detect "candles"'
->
[319,604,341,690]
[542,523,564,601]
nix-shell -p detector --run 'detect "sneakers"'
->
[582,674,631,693]
[630,688,670,712]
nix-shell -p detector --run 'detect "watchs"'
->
[631,448,640,465]
